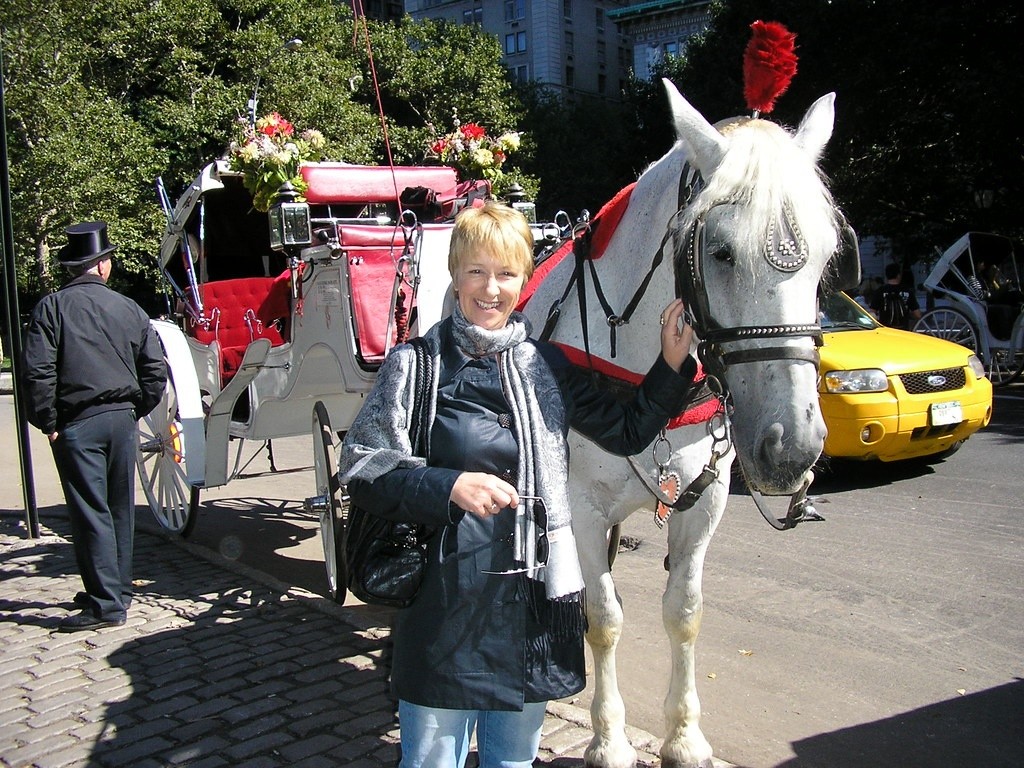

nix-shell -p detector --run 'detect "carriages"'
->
[132,78,846,768]
[910,232,1024,386]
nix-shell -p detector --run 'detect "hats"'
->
[59,220,118,266]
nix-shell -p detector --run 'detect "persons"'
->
[966,259,1024,341]
[19,220,168,632]
[851,262,922,330]
[335,199,694,768]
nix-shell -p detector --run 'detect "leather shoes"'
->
[73,592,131,609]
[58,608,125,632]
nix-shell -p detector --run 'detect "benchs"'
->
[296,164,561,261]
[987,282,1024,316]
[175,277,290,403]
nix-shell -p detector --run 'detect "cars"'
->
[817,282,992,462]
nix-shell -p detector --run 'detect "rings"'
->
[660,317,665,325]
[485,503,497,511]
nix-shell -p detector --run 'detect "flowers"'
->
[224,103,326,212]
[422,106,521,185]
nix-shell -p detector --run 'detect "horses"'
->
[442,75,854,768]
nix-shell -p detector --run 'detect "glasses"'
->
[482,495,550,575]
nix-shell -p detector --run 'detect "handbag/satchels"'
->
[343,337,432,609]
